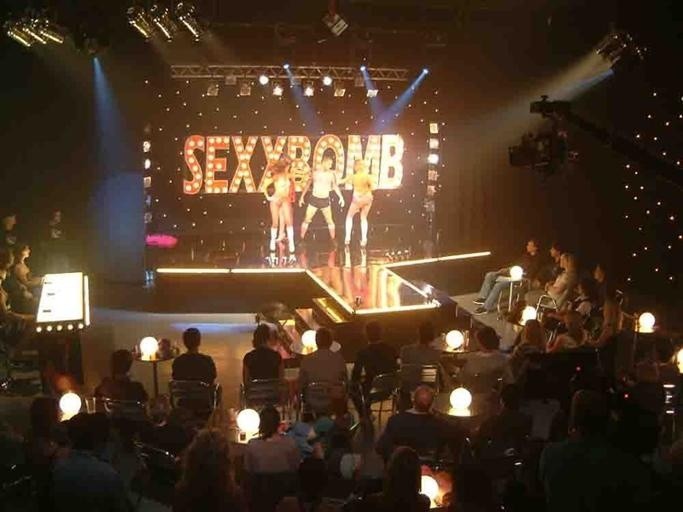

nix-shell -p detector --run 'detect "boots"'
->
[270,226,295,253]
[344,219,368,246]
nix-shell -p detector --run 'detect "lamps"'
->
[122,0,215,46]
[0,0,68,53]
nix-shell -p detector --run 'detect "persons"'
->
[45,206,69,275]
[1,317,683,512]
[337,160,375,246]
[276,204,288,242]
[507,252,578,325]
[585,298,618,345]
[497,241,568,319]
[576,263,610,308]
[541,279,600,326]
[299,157,345,241]
[0,209,54,374]
[262,159,297,254]
[471,238,543,314]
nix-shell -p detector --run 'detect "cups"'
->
[131,343,139,357]
[84,397,96,414]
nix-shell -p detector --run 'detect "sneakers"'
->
[328,238,339,247]
[473,298,486,304]
[297,238,306,246]
[474,306,488,315]
